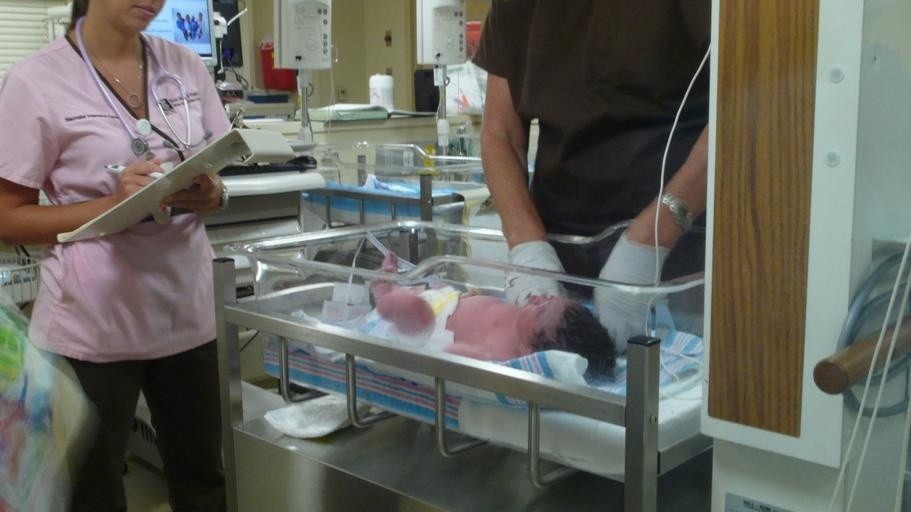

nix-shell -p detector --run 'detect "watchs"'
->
[218,184,229,211]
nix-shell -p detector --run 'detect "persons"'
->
[1,0,236,511]
[370,252,616,386]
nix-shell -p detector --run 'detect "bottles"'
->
[368,73,396,113]
[465,19,483,60]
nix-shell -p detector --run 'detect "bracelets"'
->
[657,191,695,234]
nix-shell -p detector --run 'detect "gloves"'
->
[592,231,673,355]
[504,241,568,304]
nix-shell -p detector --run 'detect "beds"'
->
[240,218,712,491]
[300,142,492,229]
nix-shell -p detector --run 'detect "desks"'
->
[246,112,541,186]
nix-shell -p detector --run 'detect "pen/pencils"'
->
[105,164,166,179]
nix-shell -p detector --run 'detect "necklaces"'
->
[472,1,711,358]
[85,35,143,108]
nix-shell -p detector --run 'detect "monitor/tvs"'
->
[141,0,217,69]
